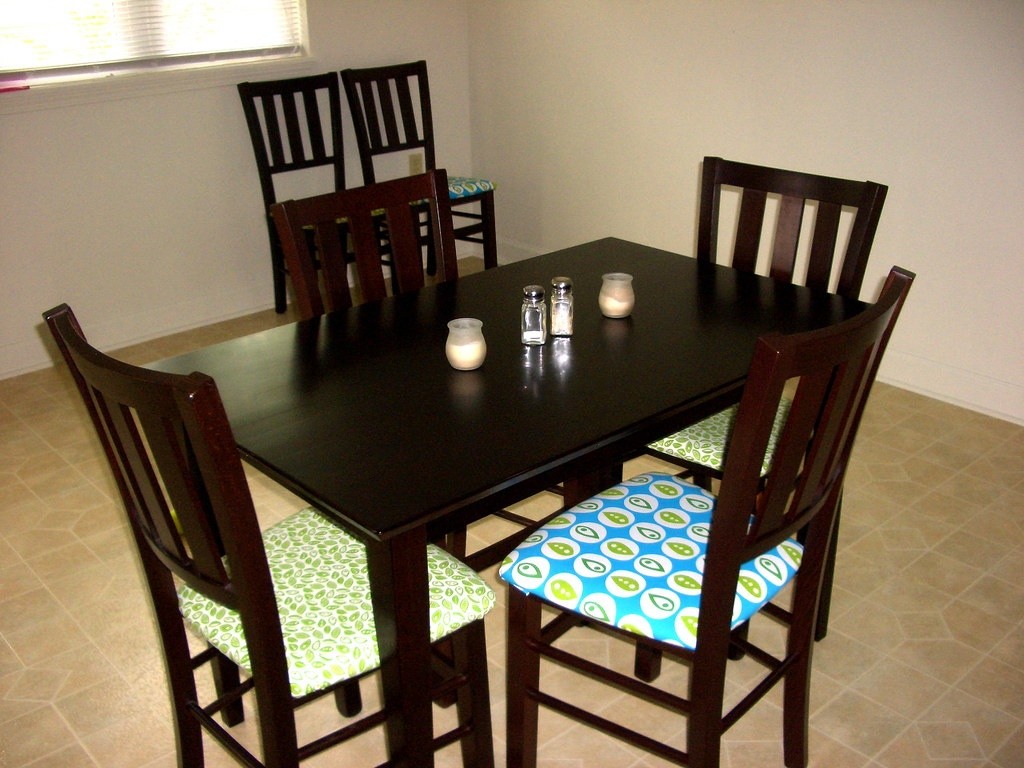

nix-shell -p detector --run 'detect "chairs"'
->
[268,169,575,576]
[644,157,890,660]
[236,59,498,313]
[42,302,498,768]
[496,266,918,768]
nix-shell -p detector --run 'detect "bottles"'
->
[446,318,486,370]
[598,273,635,318]
[550,278,574,335]
[520,286,547,344]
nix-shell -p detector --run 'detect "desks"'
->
[138,236,873,768]
